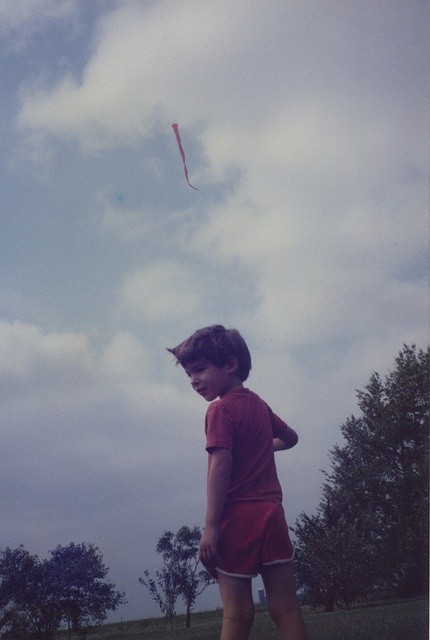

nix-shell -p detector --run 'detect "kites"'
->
[171,123,198,191]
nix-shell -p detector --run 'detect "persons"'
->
[166,325,310,640]
